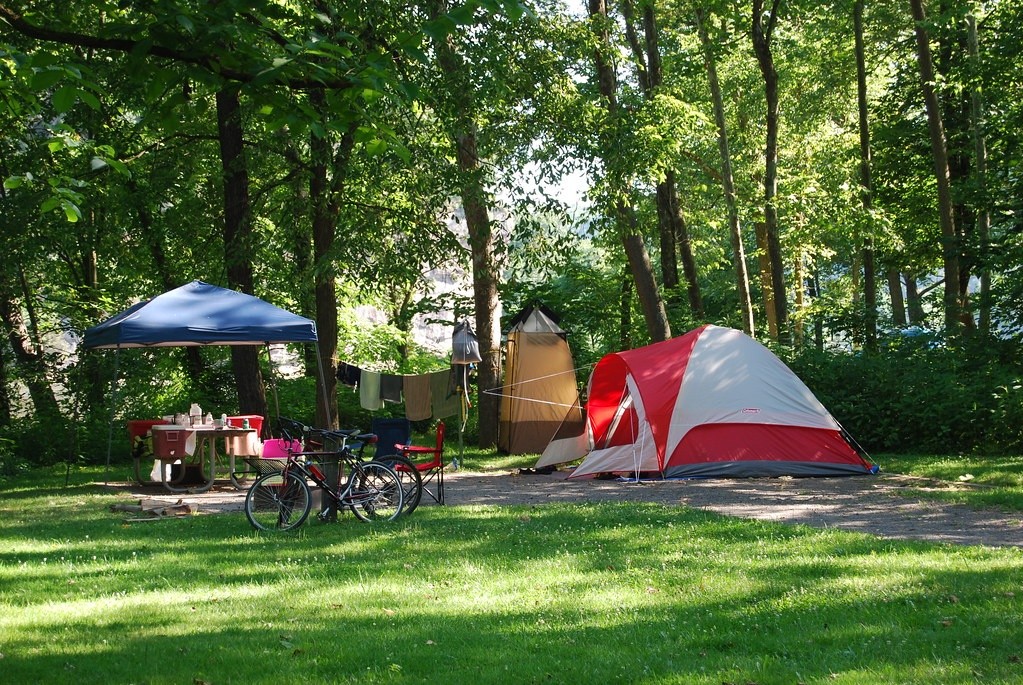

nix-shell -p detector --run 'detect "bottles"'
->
[242,418,250,429]
[306,461,326,481]
[190,403,202,428]
[175,413,183,426]
[205,412,213,428]
[183,413,190,427]
[221,413,231,427]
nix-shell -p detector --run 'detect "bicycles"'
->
[245,416,422,535]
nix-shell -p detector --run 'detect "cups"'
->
[162,415,174,424]
[214,419,224,428]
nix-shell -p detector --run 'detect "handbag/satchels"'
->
[450,326,481,365]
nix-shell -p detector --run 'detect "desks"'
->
[185,425,255,486]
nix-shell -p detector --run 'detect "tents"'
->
[499,306,584,456]
[66,279,332,491]
[531,325,878,483]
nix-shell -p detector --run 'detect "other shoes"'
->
[518,468,534,475]
[536,467,553,475]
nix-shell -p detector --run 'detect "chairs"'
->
[260,437,306,481]
[369,419,446,507]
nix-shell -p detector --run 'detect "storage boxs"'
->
[226,415,264,437]
[128,420,168,455]
[152,425,186,458]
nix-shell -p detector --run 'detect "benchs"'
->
[127,455,263,494]
